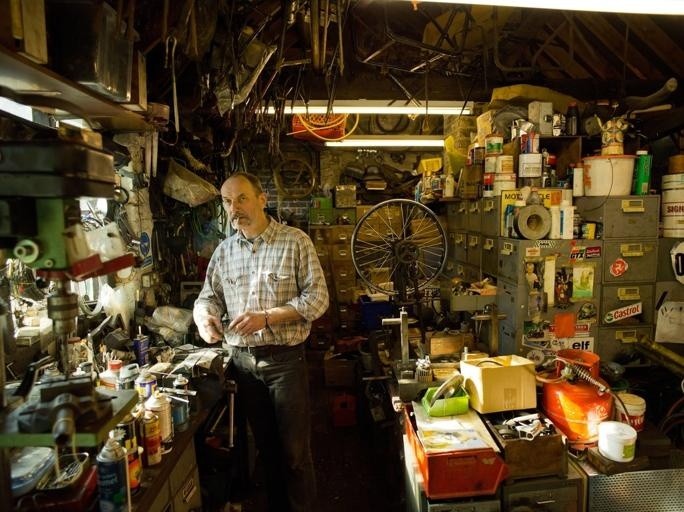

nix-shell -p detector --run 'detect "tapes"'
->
[513,204,552,239]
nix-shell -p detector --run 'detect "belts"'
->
[236,345,301,357]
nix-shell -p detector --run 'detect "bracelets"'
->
[264,307,269,330]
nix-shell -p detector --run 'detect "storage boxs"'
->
[307,180,683,512]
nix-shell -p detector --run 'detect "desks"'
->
[135,407,208,511]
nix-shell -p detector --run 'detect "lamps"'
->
[253,96,476,118]
[321,131,446,151]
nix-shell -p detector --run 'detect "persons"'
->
[192,170,329,512]
